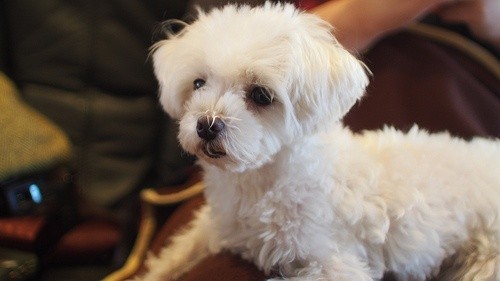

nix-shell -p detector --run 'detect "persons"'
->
[302,0,500,55]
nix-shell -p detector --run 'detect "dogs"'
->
[130,0,500,281]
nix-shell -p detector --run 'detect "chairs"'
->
[101,23,500,281]
